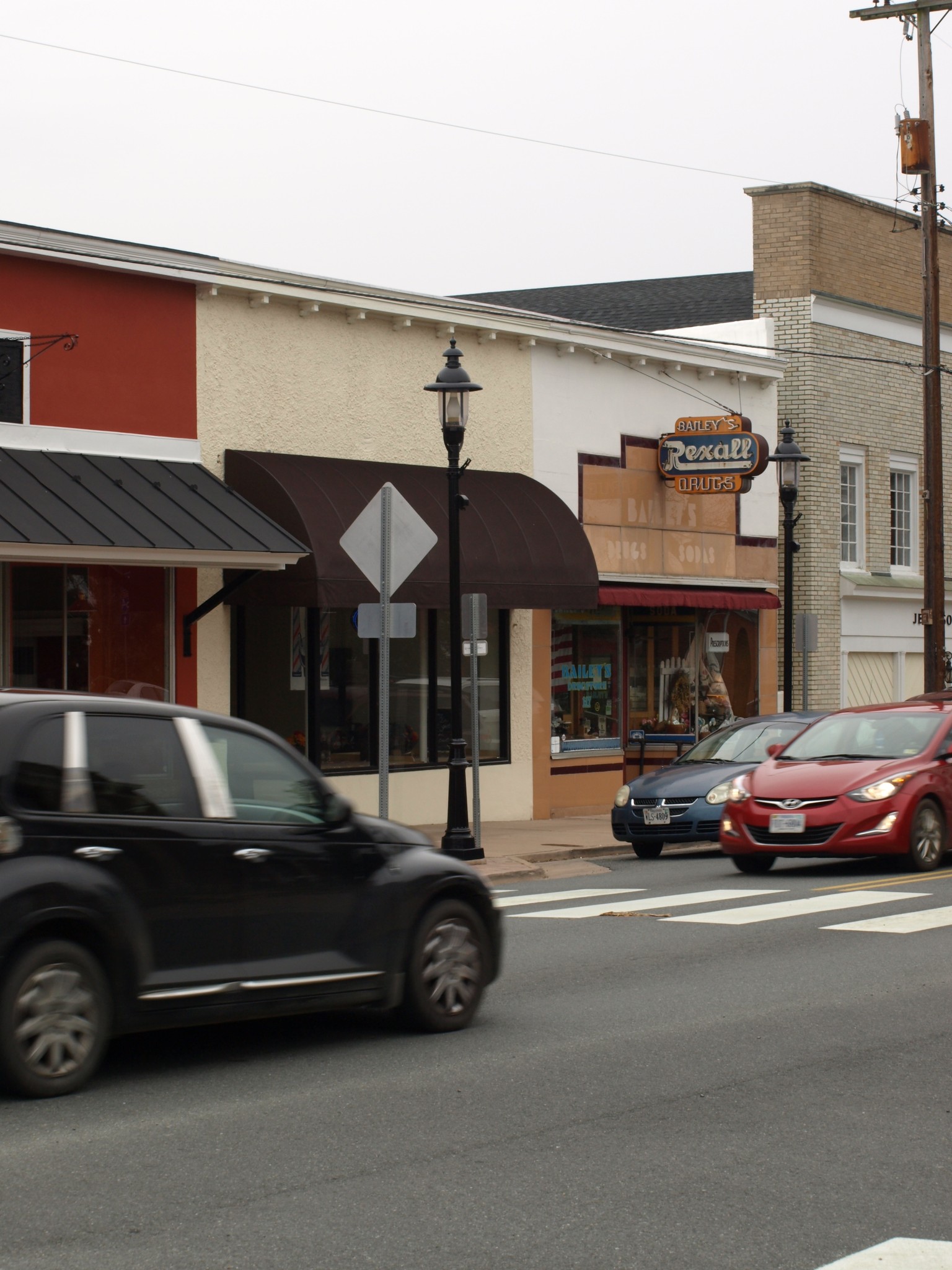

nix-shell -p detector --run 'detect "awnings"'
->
[1,445,313,572]
[221,447,599,611]
[597,572,783,610]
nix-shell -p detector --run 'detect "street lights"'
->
[765,419,811,713]
[423,336,485,859]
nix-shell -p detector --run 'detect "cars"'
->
[0,692,504,1098]
[611,711,837,858]
[718,700,951,871]
